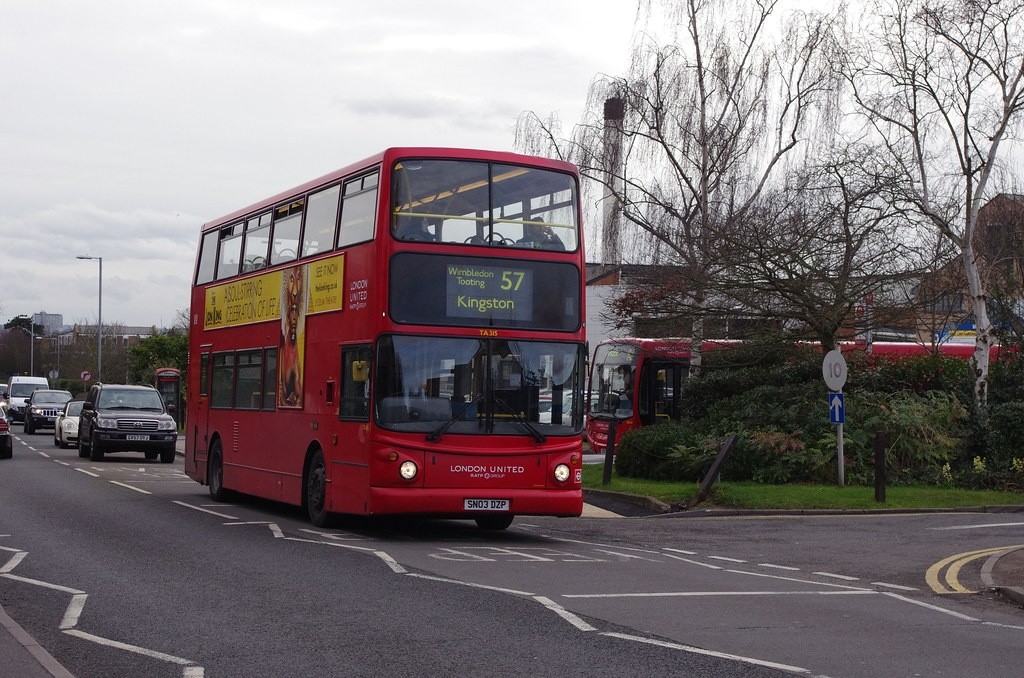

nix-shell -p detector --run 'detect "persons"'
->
[402,216,433,241]
[515,216,566,250]
[278,305,303,408]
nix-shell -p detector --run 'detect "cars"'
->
[538,388,673,428]
[0,384,12,458]
[55,401,86,448]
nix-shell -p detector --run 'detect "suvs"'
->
[24,388,73,435]
[77,381,178,463]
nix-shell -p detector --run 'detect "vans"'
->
[3,375,50,424]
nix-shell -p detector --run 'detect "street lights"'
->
[76,254,102,382]
[35,337,59,378]
[19,315,33,377]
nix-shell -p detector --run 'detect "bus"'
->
[584,337,1019,454]
[183,147,591,530]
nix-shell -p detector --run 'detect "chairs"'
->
[244,248,295,272]
